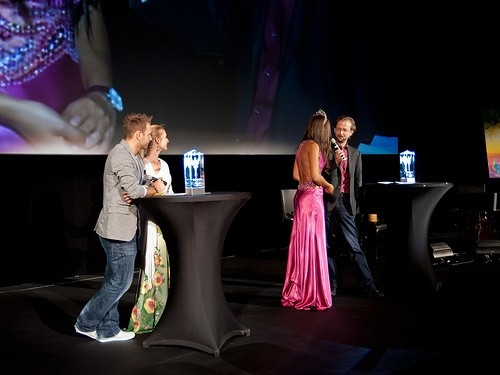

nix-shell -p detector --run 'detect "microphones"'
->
[146,175,167,186]
[332,138,346,160]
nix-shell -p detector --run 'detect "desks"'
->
[132,192,250,356]
[362,182,454,296]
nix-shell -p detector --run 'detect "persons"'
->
[0,0,123,154]
[74,114,153,342]
[322,117,384,298]
[281,109,334,310]
[123,125,173,334]
[129,0,372,152]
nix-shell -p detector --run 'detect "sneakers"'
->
[74,324,135,342]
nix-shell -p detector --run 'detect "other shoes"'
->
[364,288,384,297]
[331,281,336,295]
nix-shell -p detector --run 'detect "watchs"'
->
[87,85,123,113]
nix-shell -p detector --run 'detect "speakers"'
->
[428,242,454,259]
